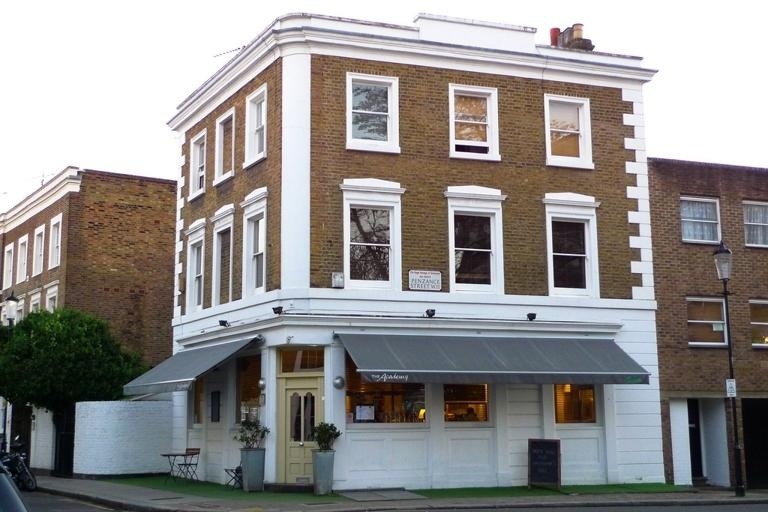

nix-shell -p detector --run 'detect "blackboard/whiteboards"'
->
[527,439,561,485]
[356,406,374,420]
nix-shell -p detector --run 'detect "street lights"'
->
[5,291,19,431]
[714,240,742,497]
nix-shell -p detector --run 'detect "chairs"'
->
[177,447,200,480]
[224,461,242,489]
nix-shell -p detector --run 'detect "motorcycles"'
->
[0,434,36,492]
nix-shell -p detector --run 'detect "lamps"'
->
[219,320,228,326]
[527,313,536,321]
[272,306,283,314]
[426,309,435,317]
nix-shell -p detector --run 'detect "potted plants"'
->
[232,417,270,491]
[306,422,343,496]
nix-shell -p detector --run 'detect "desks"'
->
[160,453,189,483]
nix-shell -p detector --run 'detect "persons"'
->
[458,407,482,421]
